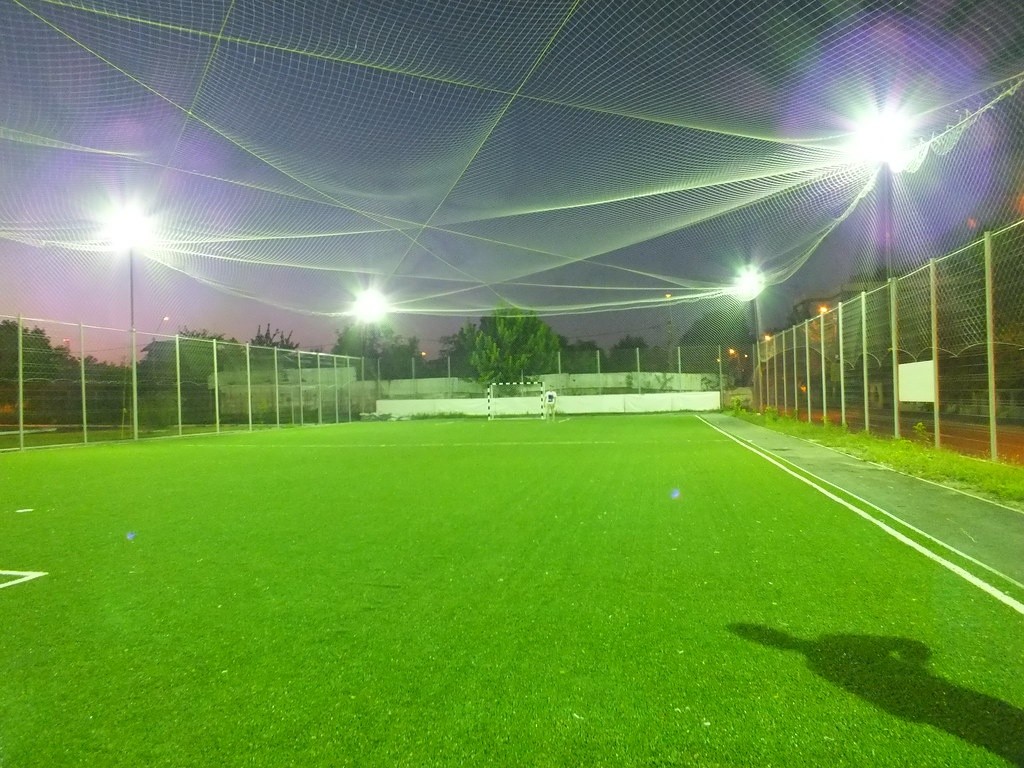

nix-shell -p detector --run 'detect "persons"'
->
[543,385,557,420]
